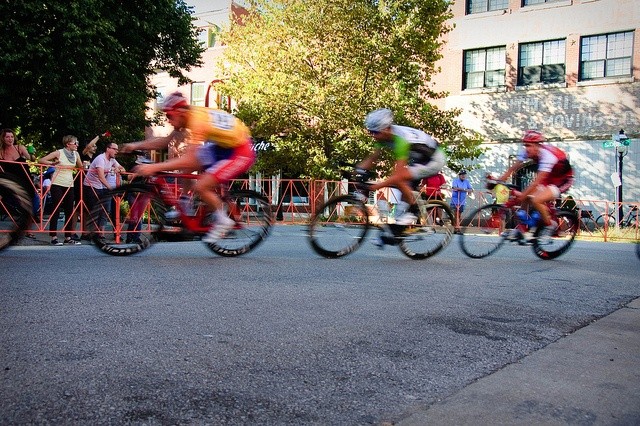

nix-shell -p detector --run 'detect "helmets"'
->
[159,92,188,111]
[367,109,392,131]
[522,130,545,142]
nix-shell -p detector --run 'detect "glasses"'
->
[165,113,174,119]
[370,130,378,134]
[68,142,79,145]
[109,147,118,150]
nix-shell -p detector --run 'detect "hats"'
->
[458,170,467,175]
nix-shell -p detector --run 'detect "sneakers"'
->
[380,233,401,245]
[398,213,418,225]
[24,234,35,239]
[454,230,463,235]
[51,238,63,246]
[64,239,81,245]
[80,235,93,241]
[165,195,194,219]
[202,210,235,242]
[543,220,557,241]
[508,230,523,241]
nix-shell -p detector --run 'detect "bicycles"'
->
[308,171,454,261]
[1,175,33,252]
[597,203,640,230]
[569,206,596,232]
[90,152,273,257]
[459,178,580,260]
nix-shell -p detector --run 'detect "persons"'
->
[419,174,448,224]
[499,128,574,245]
[38,134,88,247]
[483,181,510,234]
[125,147,152,244]
[352,108,447,228]
[440,186,442,189]
[82,132,106,162]
[116,90,258,242]
[41,167,66,222]
[450,168,473,233]
[0,127,42,239]
[83,142,127,243]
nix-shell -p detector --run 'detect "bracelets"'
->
[119,169,121,173]
[98,133,102,139]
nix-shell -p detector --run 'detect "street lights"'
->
[613,129,630,231]
